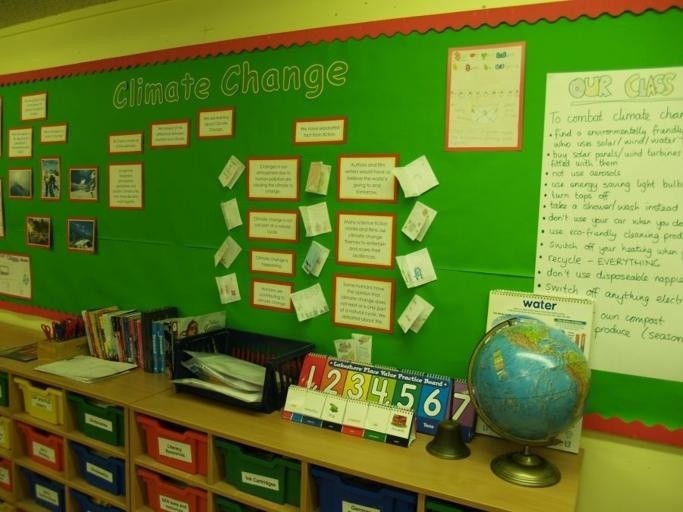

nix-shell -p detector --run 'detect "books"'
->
[79,303,228,381]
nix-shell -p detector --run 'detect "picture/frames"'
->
[443,39,523,152]
[107,161,144,210]
[332,274,396,333]
[196,106,236,139]
[246,156,302,312]
[8,92,100,254]
[293,114,347,145]
[148,119,191,149]
[336,154,401,204]
[109,130,144,152]
[334,211,396,270]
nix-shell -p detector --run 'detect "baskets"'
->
[174,328,314,413]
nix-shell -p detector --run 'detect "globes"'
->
[467,315,593,489]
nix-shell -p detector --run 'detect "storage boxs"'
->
[13,421,64,475]
[132,467,208,511]
[26,473,64,510]
[306,463,420,510]
[10,376,67,430]
[69,442,126,498]
[134,414,208,479]
[73,397,126,452]
[1,416,13,452]
[1,461,13,495]
[211,433,299,508]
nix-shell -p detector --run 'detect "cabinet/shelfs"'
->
[0,319,176,511]
[131,373,584,511]
